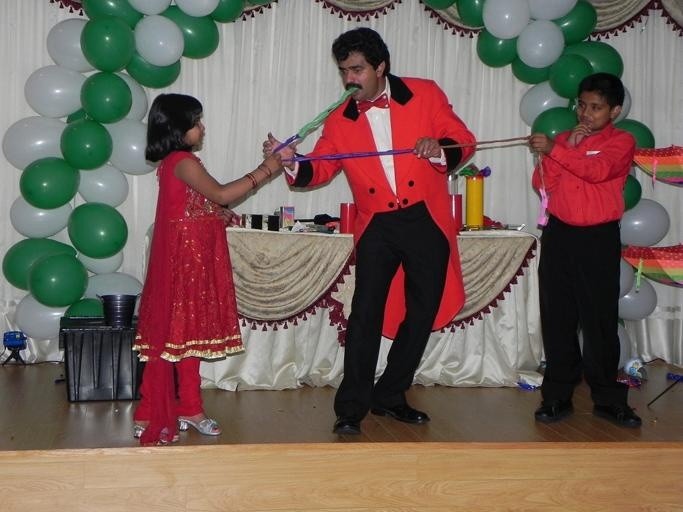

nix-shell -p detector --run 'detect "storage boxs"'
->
[56,316,142,403]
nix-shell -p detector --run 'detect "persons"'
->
[133,94,283,446]
[527,72,642,429]
[262,28,476,436]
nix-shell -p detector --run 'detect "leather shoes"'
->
[333,414,362,436]
[593,401,640,427]
[532,398,573,422]
[371,397,430,423]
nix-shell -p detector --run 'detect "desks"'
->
[198,226,546,392]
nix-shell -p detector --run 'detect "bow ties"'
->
[355,93,388,115]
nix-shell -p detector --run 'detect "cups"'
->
[448,193,462,236]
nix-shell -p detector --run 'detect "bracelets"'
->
[245,163,272,188]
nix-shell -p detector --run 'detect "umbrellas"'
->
[622,245,683,288]
[633,144,683,187]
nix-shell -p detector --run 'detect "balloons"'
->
[421,1,656,167]
[577,173,671,371]
[0,0,275,341]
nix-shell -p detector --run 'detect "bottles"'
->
[447,174,458,196]
[339,202,356,234]
[465,175,484,229]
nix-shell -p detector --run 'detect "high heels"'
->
[177,415,221,436]
[132,419,178,443]
